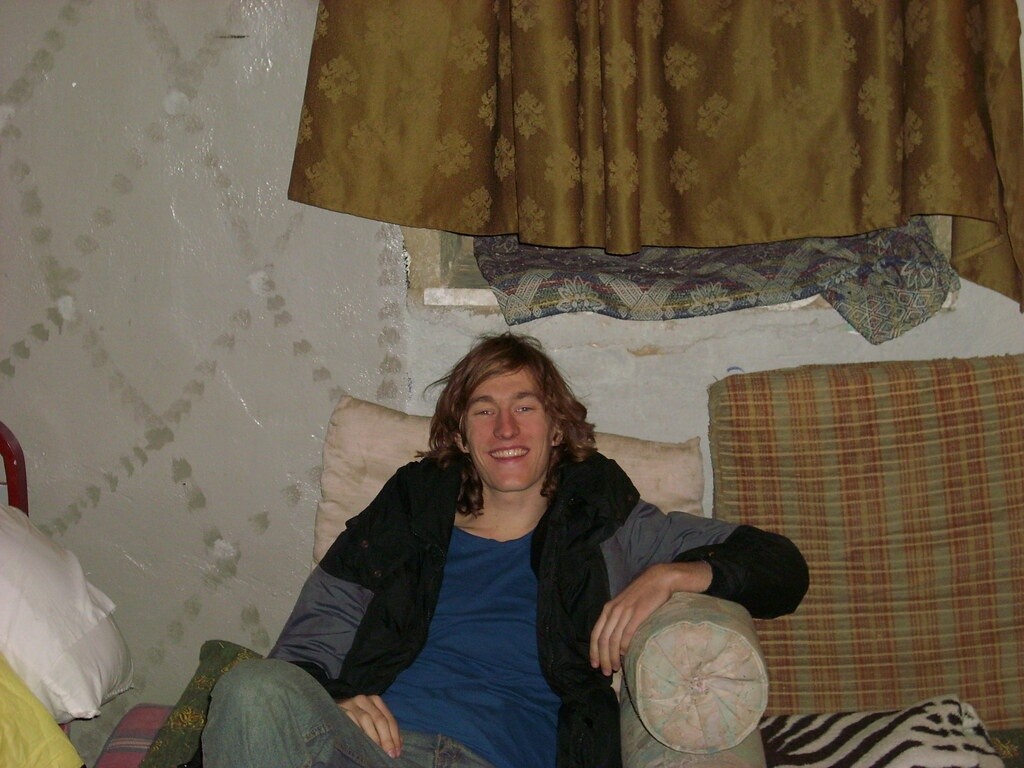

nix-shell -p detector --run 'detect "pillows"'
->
[309,391,704,569]
[0,501,136,722]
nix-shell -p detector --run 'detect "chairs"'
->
[134,360,1023,768]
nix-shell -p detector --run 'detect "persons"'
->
[200,333,810,768]
